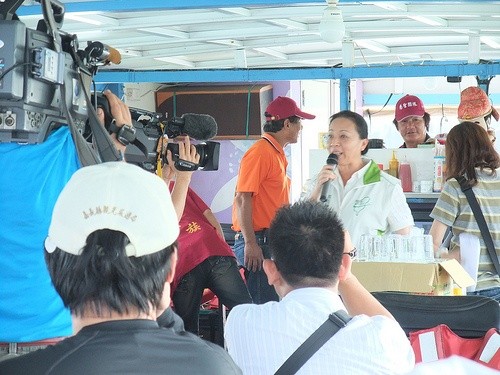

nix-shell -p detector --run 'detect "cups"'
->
[355,233,435,262]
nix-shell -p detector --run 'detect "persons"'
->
[153,134,256,339]
[1,87,133,361]
[1,160,246,375]
[300,110,415,255]
[394,93,435,149]
[223,198,415,375]
[234,95,316,304]
[458,87,500,151]
[426,121,500,309]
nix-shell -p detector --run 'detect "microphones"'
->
[91,42,121,65]
[172,113,217,140]
[320,154,339,202]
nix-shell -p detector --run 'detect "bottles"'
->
[389,151,399,177]
[377,163,383,173]
[433,145,446,192]
[399,155,413,194]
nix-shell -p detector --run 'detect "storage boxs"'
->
[345,257,478,296]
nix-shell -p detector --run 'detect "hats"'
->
[457,86,499,123]
[395,94,424,122]
[44,160,181,258]
[265,96,316,122]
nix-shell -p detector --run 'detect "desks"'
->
[403,188,447,225]
